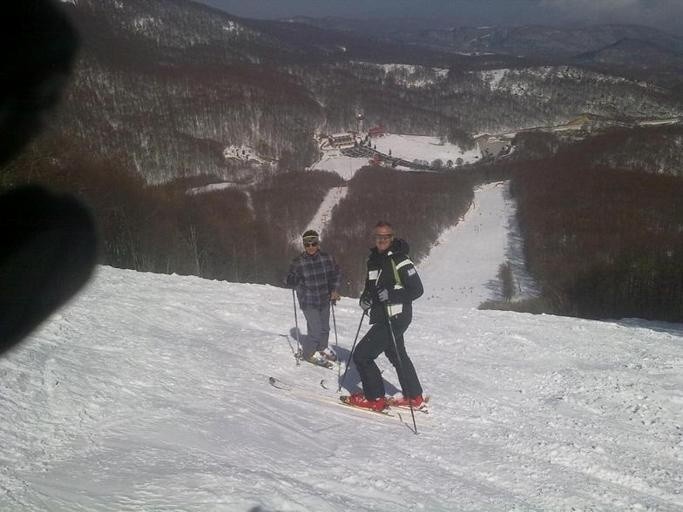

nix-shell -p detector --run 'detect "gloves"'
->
[328,290,342,306]
[376,288,389,303]
[359,296,373,311]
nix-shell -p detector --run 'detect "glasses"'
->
[372,234,394,242]
[304,243,318,247]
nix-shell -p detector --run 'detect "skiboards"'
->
[269,377,441,429]
[293,348,352,372]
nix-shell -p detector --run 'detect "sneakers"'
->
[318,347,337,361]
[305,349,328,366]
[385,393,423,408]
[349,391,387,412]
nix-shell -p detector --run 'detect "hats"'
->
[302,230,320,243]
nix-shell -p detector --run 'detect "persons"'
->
[286,228,340,370]
[349,220,424,412]
